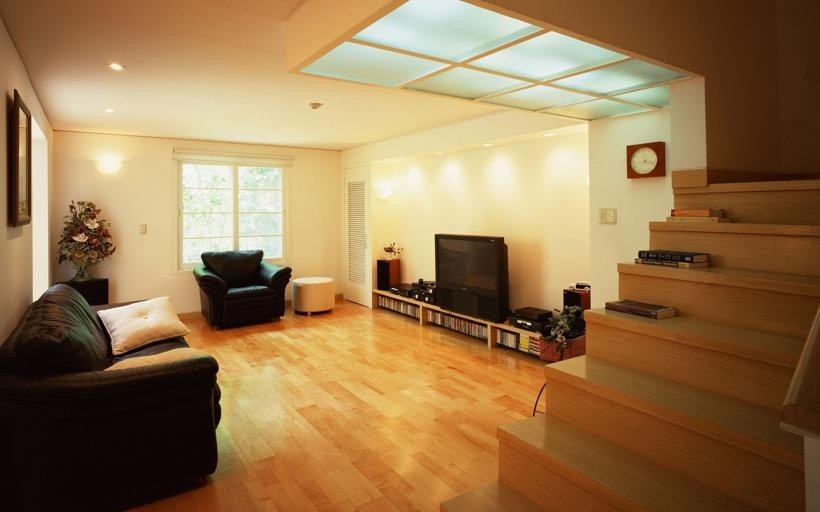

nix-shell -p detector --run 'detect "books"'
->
[605,299,677,320]
[633,248,710,270]
[378,296,540,357]
[666,205,734,224]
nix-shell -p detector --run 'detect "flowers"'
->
[54,200,117,268]
[541,306,587,354]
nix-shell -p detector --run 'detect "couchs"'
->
[0,283,224,512]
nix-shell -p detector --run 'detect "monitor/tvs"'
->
[435,233,508,298]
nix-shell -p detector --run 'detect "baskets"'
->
[540,335,585,363]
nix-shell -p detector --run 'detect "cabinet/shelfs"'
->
[342,163,398,308]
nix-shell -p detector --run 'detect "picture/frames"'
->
[8,88,36,228]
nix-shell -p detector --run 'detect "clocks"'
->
[626,141,667,180]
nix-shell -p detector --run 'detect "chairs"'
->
[192,248,293,328]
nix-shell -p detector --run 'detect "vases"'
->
[68,257,93,282]
[540,333,587,361]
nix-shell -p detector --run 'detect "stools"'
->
[291,277,335,317]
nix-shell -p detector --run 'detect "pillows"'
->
[95,296,191,356]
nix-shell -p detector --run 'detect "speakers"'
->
[377,258,400,291]
[564,288,591,335]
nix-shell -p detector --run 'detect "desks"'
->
[55,277,109,304]
[373,287,540,350]
